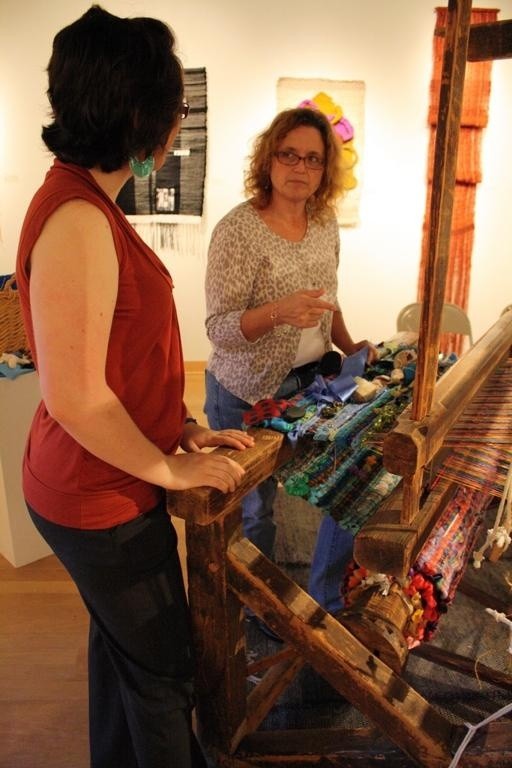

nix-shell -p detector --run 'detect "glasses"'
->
[158,103,189,120]
[273,151,327,170]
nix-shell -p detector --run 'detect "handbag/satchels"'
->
[1,273,32,352]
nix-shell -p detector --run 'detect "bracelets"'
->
[270,303,281,326]
[183,417,197,427]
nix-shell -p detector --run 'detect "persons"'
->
[197,102,382,617]
[13,3,261,768]
